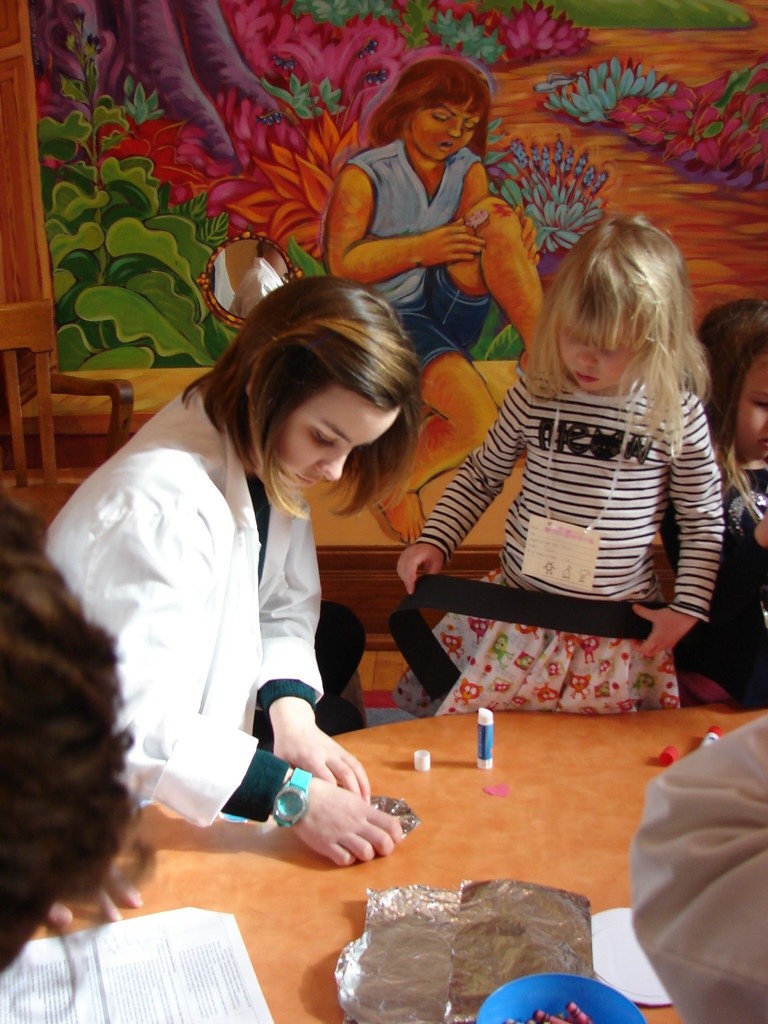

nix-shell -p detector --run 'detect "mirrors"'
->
[198,231,304,329]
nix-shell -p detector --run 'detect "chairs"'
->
[0,298,79,527]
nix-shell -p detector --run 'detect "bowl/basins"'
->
[476,973,646,1024]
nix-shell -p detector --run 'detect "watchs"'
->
[274,768,312,827]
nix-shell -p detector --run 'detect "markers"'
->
[698,724,721,749]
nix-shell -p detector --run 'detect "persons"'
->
[48,275,421,864]
[659,299,768,707]
[394,219,725,714]
[630,714,768,1024]
[0,496,143,969]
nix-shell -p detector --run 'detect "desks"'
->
[24,705,768,1023]
